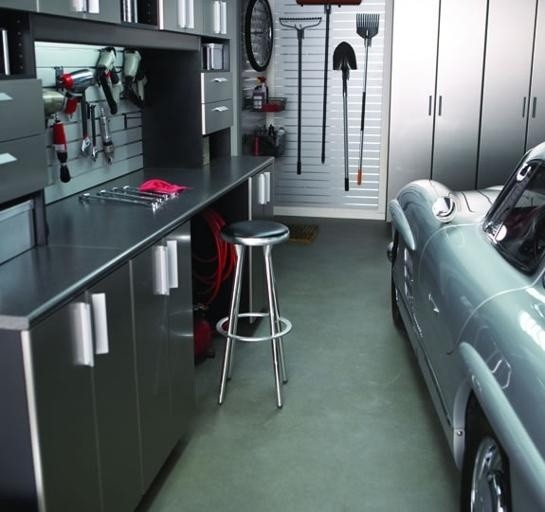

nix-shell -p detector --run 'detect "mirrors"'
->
[244,0,274,73]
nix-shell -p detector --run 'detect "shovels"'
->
[334,42,357,191]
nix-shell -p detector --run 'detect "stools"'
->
[215,219,293,409]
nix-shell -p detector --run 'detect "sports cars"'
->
[393,139,543,507]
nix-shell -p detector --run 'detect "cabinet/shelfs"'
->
[479,1,544,188]
[200,69,234,136]
[206,163,276,325]
[129,220,196,495]
[0,0,231,43]
[1,262,145,512]
[0,78,49,204]
[387,0,488,226]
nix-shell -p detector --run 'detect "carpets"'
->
[279,222,320,247]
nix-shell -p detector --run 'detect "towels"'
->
[140,179,188,192]
